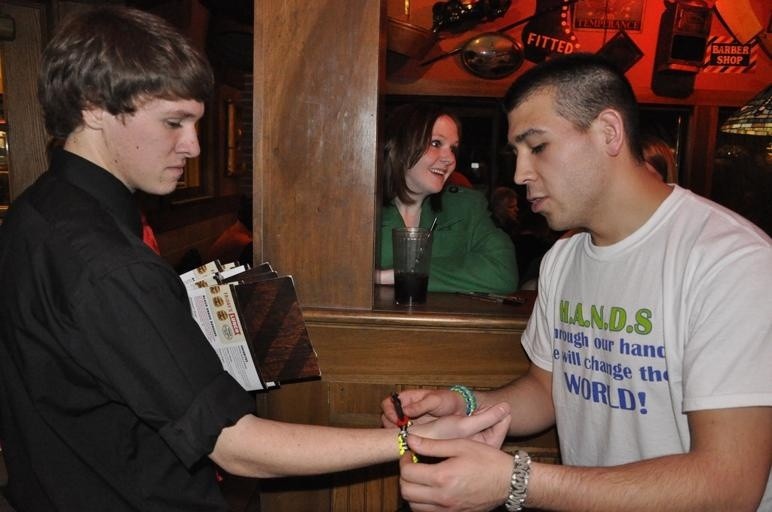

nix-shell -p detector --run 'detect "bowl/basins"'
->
[387,14,442,60]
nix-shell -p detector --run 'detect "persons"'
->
[380,52,772,510]
[375,99,674,293]
[1,1,513,511]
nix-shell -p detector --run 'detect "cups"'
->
[390,228,432,306]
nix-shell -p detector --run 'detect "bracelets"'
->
[450,384,476,416]
[504,449,532,512]
[397,423,420,464]
